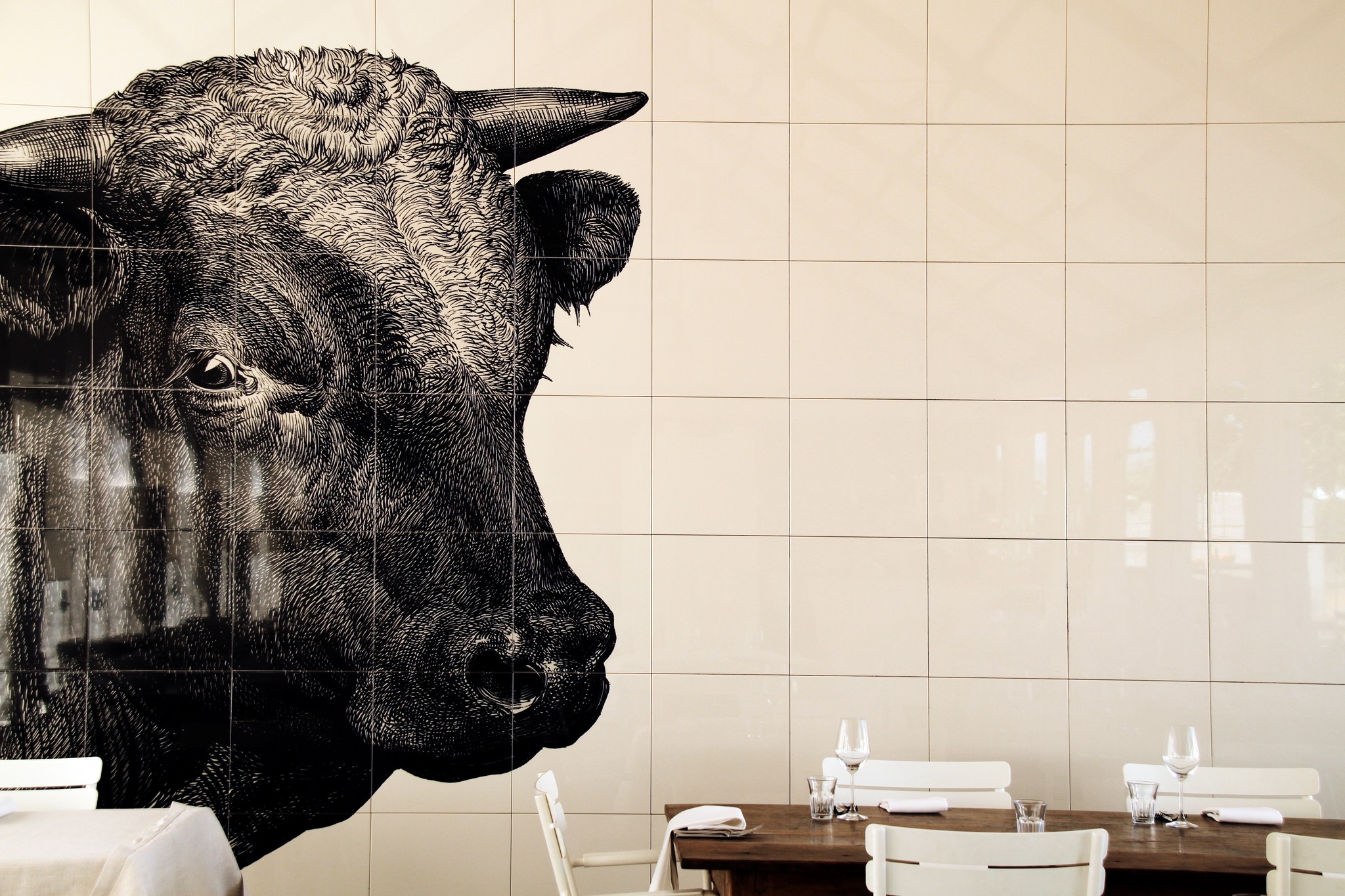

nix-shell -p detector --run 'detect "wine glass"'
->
[833,717,871,821]
[1161,724,1200,829]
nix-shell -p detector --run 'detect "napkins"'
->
[876,797,948,813]
[648,806,747,891]
[1200,807,1283,824]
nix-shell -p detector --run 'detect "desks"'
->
[0,803,244,896]
[664,804,1345,896]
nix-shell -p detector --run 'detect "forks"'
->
[1184,814,1187,819]
[674,823,765,837]
[1154,812,1163,820]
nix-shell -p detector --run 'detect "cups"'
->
[1013,799,1048,833]
[806,775,838,820]
[1127,780,1159,824]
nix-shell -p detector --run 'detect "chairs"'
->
[1123,764,1321,819]
[535,770,711,896]
[865,824,1108,896]
[823,757,1010,808]
